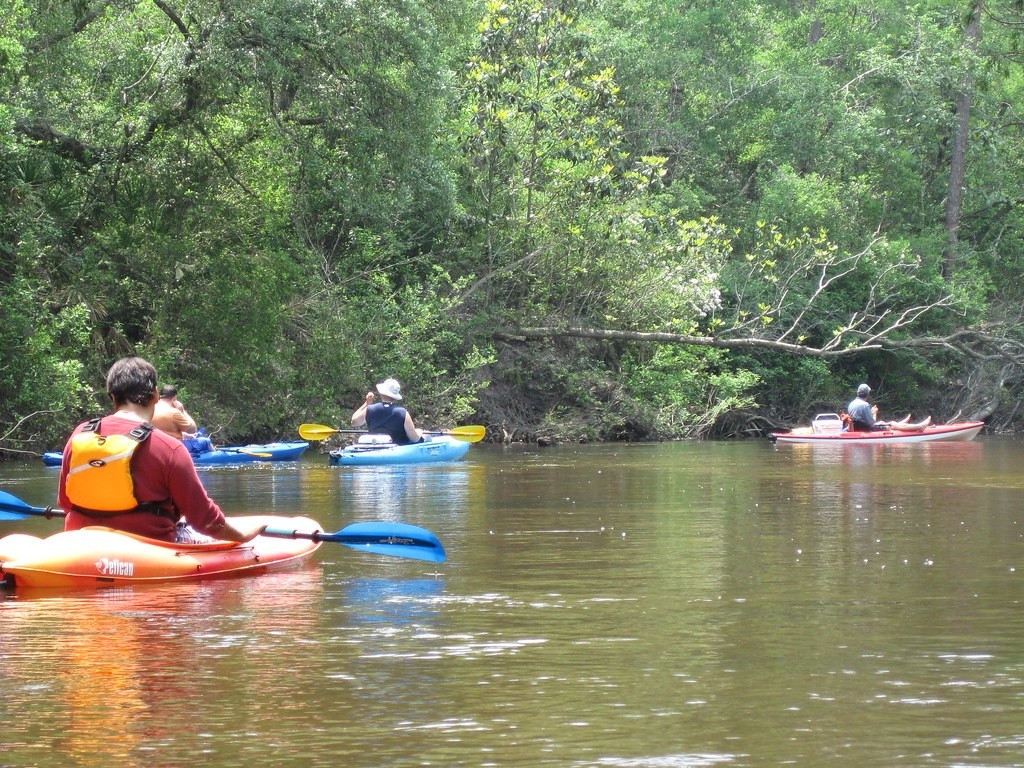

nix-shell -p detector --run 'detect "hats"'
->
[376,379,402,400]
[857,383,871,396]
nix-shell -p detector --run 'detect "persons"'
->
[153,384,217,457]
[349,378,433,447]
[54,357,271,544]
[840,383,931,434]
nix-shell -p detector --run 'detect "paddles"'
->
[297,422,488,444]
[1,488,446,565]
[212,447,274,458]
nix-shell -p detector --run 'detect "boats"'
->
[0,516,328,601]
[319,433,472,467]
[44,442,311,470]
[762,413,984,446]
